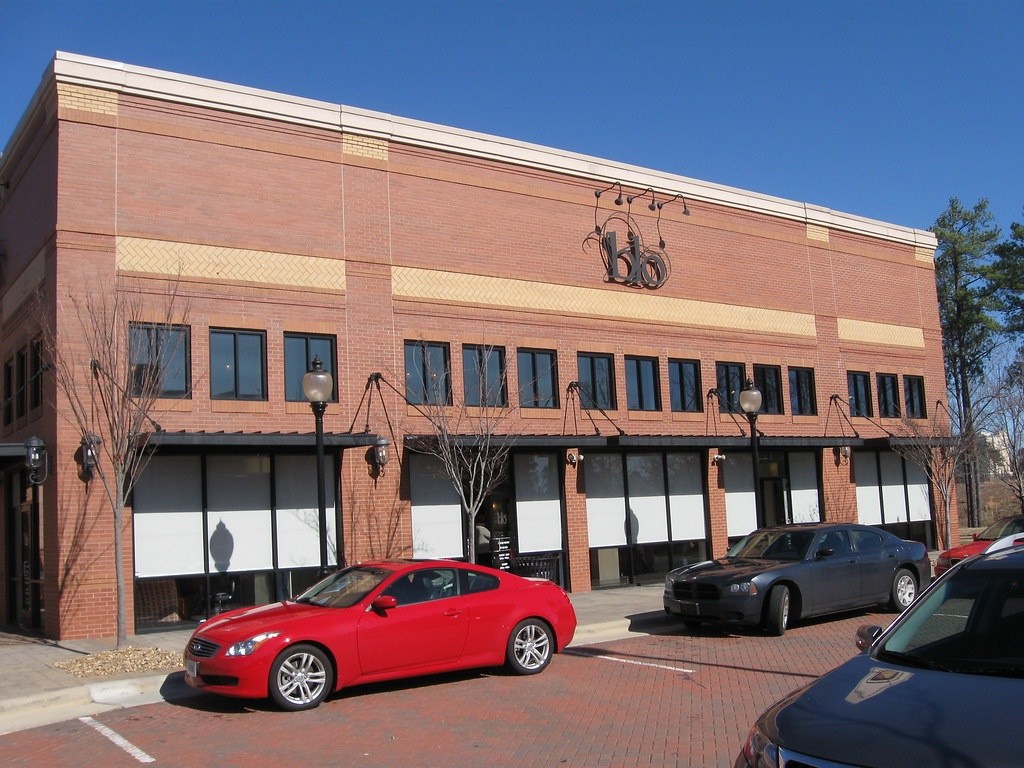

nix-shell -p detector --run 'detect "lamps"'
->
[80,430,103,479]
[839,447,850,466]
[713,454,726,463]
[656,194,690,216]
[568,453,584,466]
[373,436,392,476]
[627,188,656,212]
[594,182,624,205]
[24,436,48,485]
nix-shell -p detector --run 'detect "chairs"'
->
[408,571,439,602]
[385,576,410,606]
[134,579,187,624]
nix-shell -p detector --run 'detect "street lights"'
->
[302,355,336,581]
[738,374,764,529]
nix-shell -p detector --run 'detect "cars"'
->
[933,515,1024,578]
[183,556,578,713]
[661,522,932,637]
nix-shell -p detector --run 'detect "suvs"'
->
[732,531,1024,768]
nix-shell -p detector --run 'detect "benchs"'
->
[446,576,491,595]
